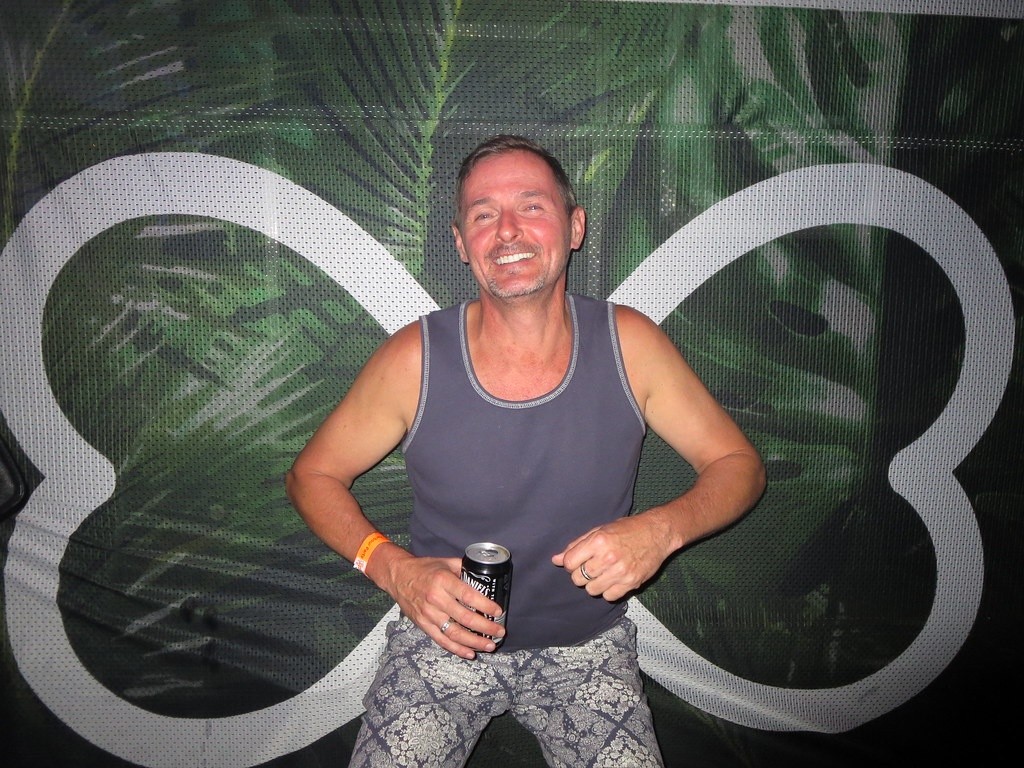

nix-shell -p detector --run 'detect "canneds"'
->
[458,542,512,648]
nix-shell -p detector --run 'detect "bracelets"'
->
[351,532,394,581]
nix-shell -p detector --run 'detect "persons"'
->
[283,132,770,768]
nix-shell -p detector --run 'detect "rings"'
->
[439,617,458,634]
[580,562,592,581]
